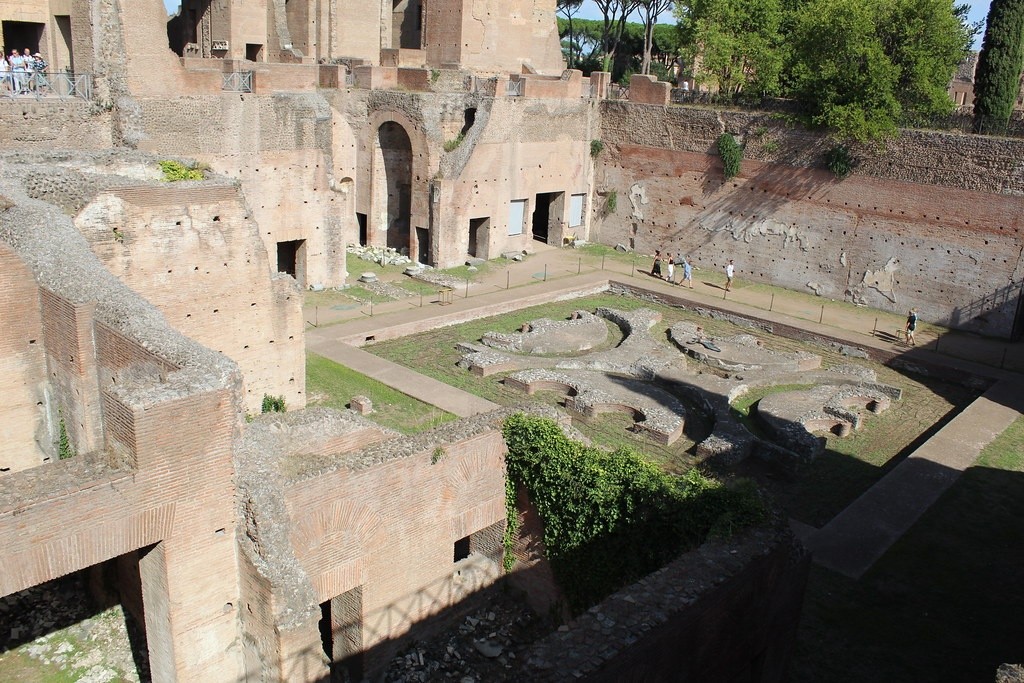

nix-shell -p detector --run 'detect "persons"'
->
[0,48,49,97]
[651,250,664,278]
[905,308,918,345]
[665,253,673,283]
[679,260,693,289]
[725,260,734,292]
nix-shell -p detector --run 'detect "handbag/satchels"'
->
[656,260,660,264]
[909,324,913,329]
